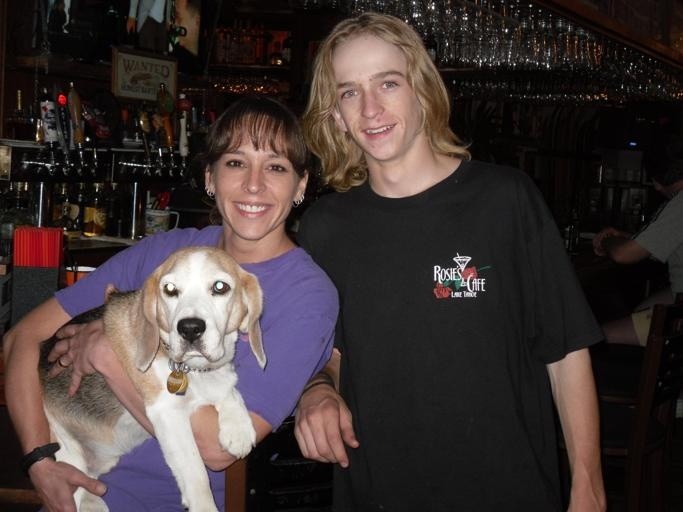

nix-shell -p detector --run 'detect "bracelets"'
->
[21,442,59,480]
[301,371,338,396]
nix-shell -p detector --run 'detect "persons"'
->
[150,109,167,150]
[136,106,153,138]
[1,93,340,511]
[291,10,606,511]
[589,128,681,348]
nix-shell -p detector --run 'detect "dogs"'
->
[37,246,266,511]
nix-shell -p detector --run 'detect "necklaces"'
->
[160,340,231,395]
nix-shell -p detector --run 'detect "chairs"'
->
[587,288,683,512]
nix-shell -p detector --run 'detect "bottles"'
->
[4,180,121,238]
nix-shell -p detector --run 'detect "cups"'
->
[143,208,180,236]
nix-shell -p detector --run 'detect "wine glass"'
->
[347,1,683,107]
[38,77,190,178]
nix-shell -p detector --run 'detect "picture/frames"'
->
[110,45,179,105]
